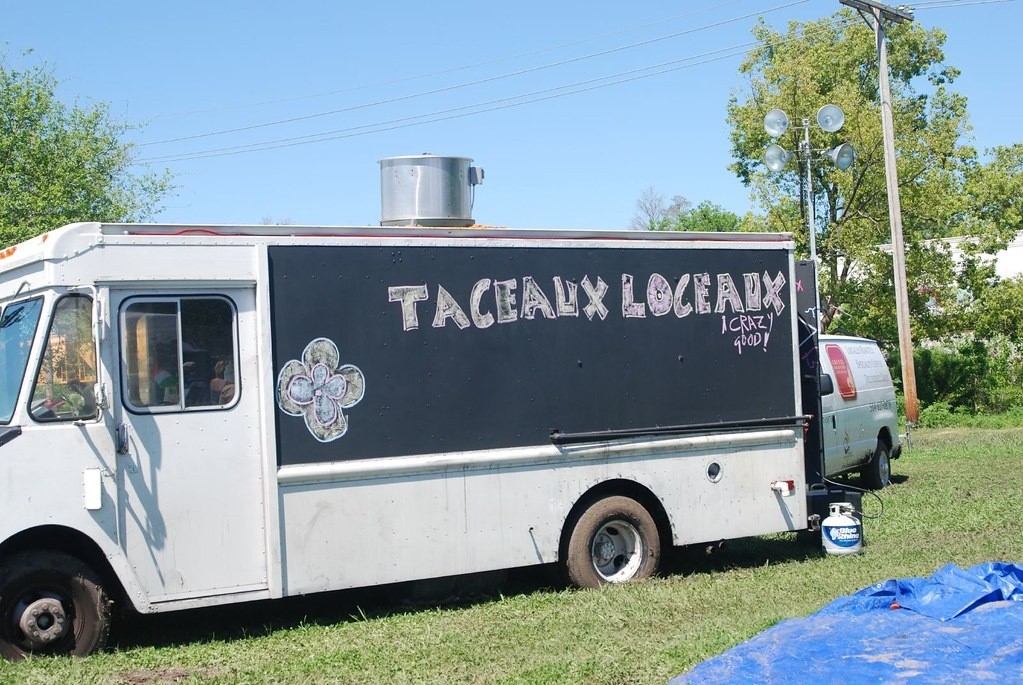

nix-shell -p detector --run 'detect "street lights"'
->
[764,103,856,261]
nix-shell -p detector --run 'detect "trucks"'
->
[0,151,825,666]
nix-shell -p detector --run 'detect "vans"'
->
[817,335,903,492]
